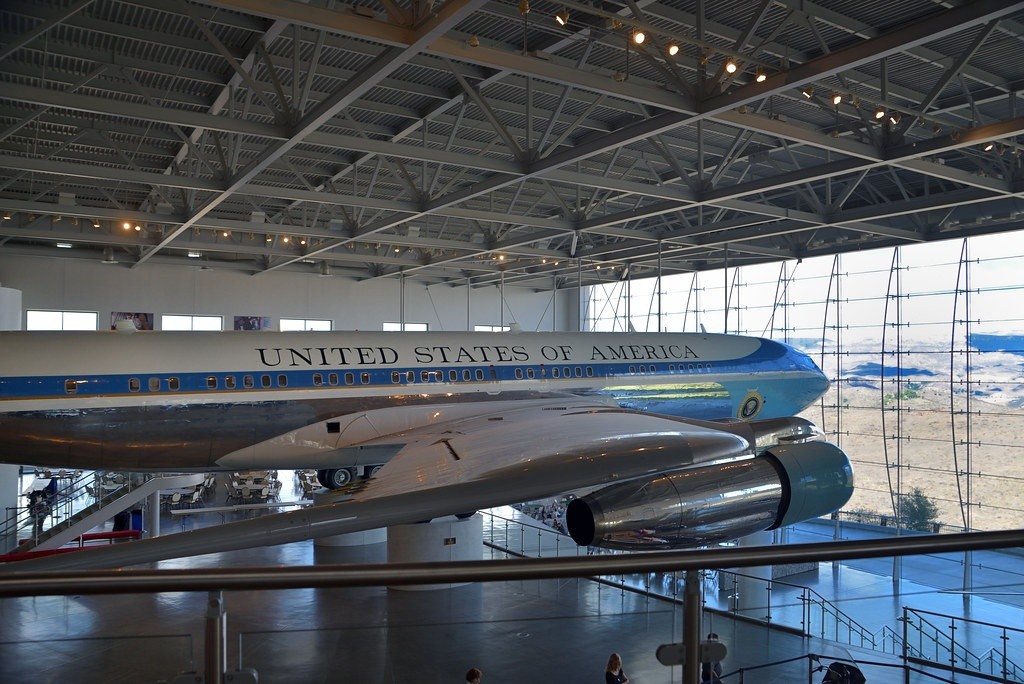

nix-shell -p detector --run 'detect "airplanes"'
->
[0,330,853,579]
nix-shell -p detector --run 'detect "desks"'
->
[309,482,322,486]
[160,489,193,499]
[235,485,266,489]
[240,475,265,479]
[305,473,317,477]
[102,484,123,489]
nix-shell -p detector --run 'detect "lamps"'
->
[555,7,570,26]
[803,86,815,100]
[983,143,993,151]
[890,111,901,124]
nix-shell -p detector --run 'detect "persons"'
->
[465,667,482,684]
[606,652,629,684]
[30,491,48,537]
[701,632,723,684]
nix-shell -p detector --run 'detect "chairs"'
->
[224,470,282,503]
[85,474,124,505]
[160,476,217,519]
[34,469,79,483]
[298,470,316,499]
